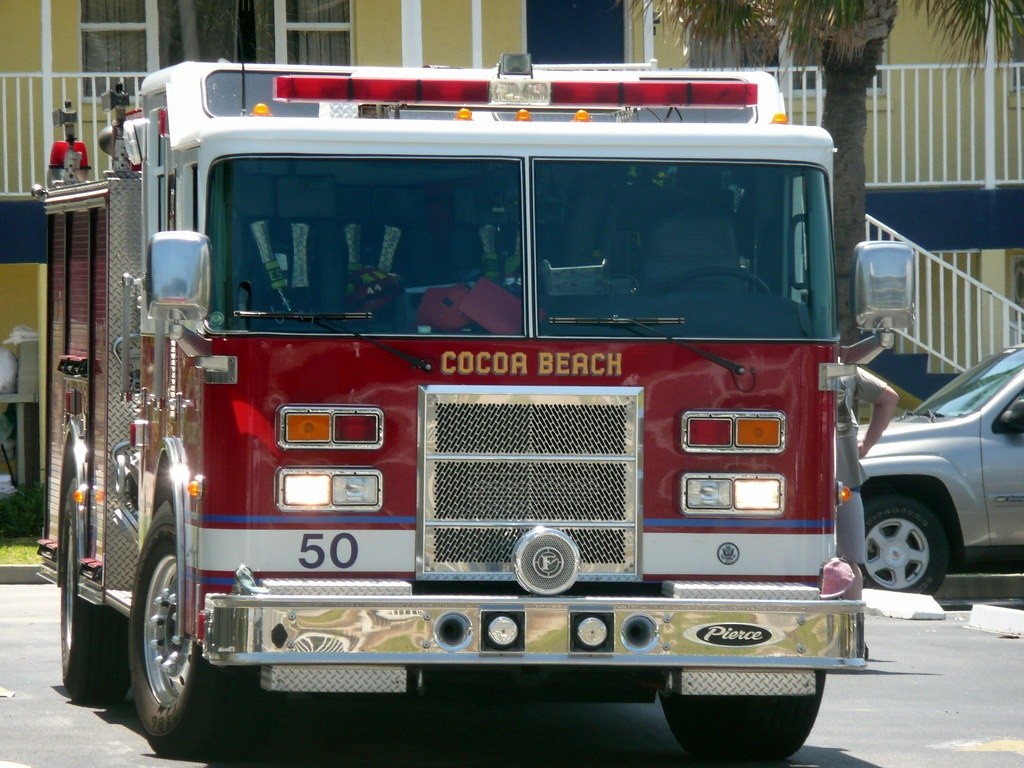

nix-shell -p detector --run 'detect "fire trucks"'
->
[47,55,916,765]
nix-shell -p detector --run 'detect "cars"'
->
[857,344,1024,599]
